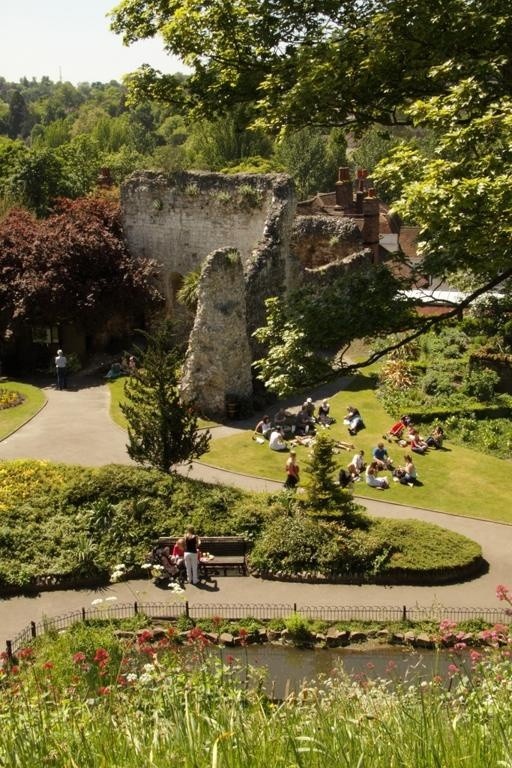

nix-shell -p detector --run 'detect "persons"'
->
[173,524,203,585]
[102,355,137,379]
[53,349,67,390]
[251,397,445,491]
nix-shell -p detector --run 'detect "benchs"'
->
[158,536,247,576]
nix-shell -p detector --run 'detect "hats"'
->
[307,397,312,403]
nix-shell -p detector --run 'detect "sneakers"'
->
[408,482,414,487]
